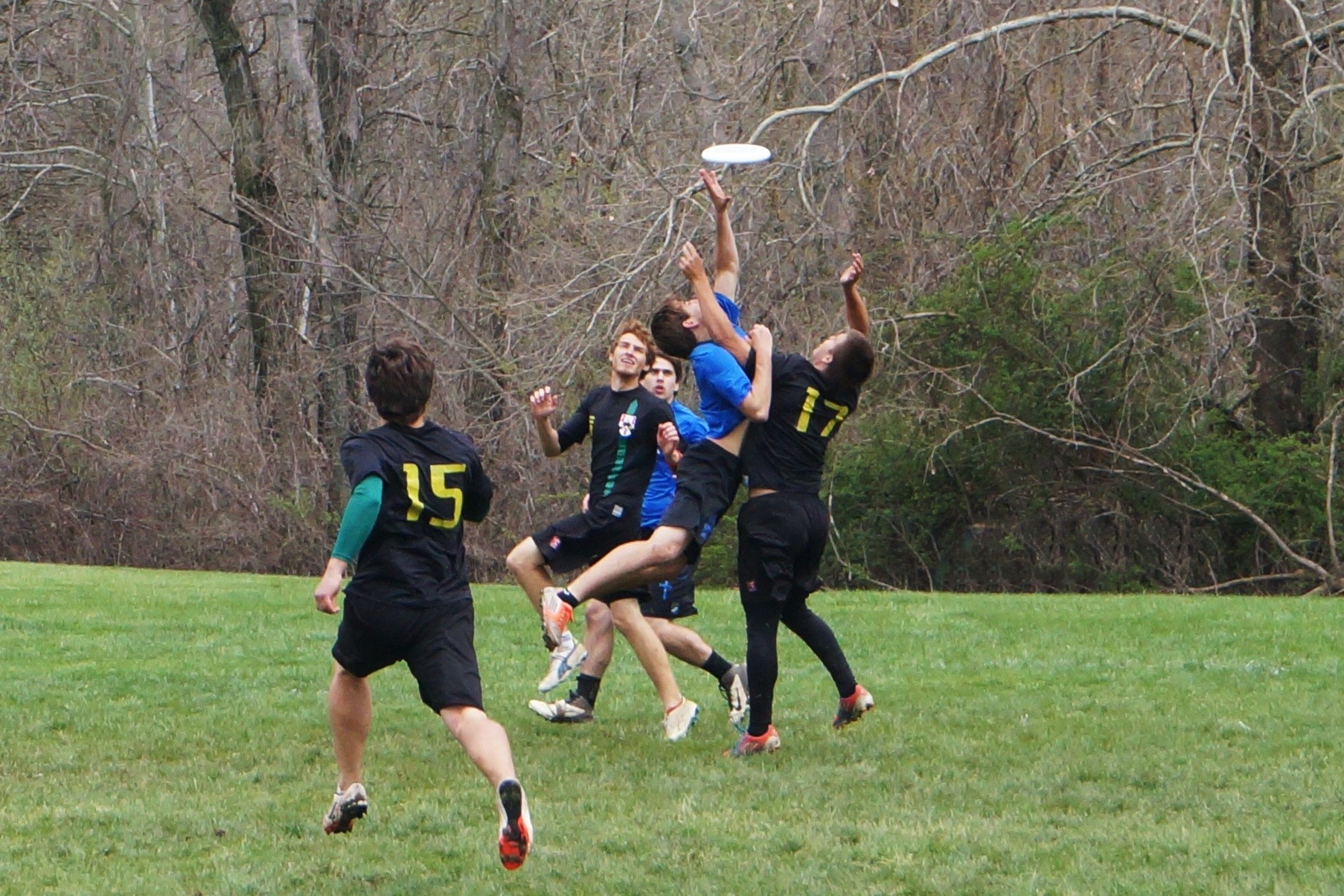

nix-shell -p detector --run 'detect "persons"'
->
[501,171,876,760]
[311,338,533,872]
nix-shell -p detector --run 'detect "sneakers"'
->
[540,587,573,651]
[497,777,533,870]
[832,684,874,729]
[661,696,700,741]
[722,714,781,759]
[537,638,587,692]
[718,662,750,724]
[323,783,368,834]
[527,690,594,723]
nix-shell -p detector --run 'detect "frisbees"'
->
[699,140,773,166]
